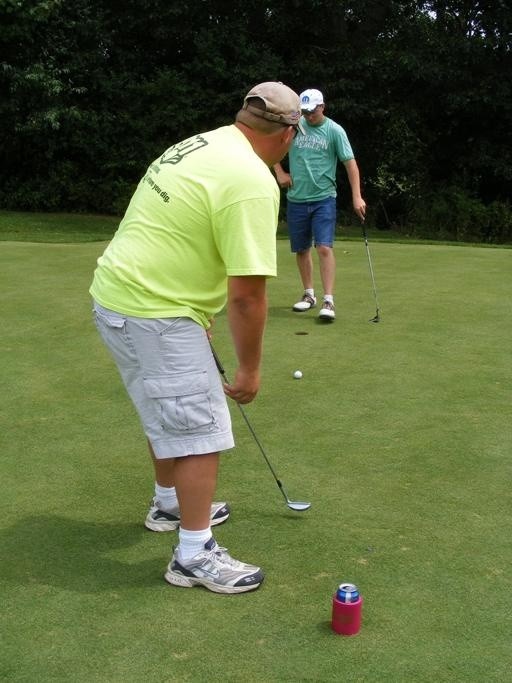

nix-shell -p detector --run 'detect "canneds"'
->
[337,583,359,602]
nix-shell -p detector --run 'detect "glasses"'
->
[291,123,300,139]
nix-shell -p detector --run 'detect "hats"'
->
[298,89,325,111]
[242,79,309,136]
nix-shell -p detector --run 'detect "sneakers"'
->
[292,294,318,312]
[163,537,264,595]
[317,300,337,321]
[143,494,231,533]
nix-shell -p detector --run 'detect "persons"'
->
[274,88,366,322]
[88,77,304,596]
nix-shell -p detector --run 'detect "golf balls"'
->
[294,370,302,379]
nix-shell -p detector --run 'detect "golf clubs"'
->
[361,217,380,322]
[208,338,311,510]
